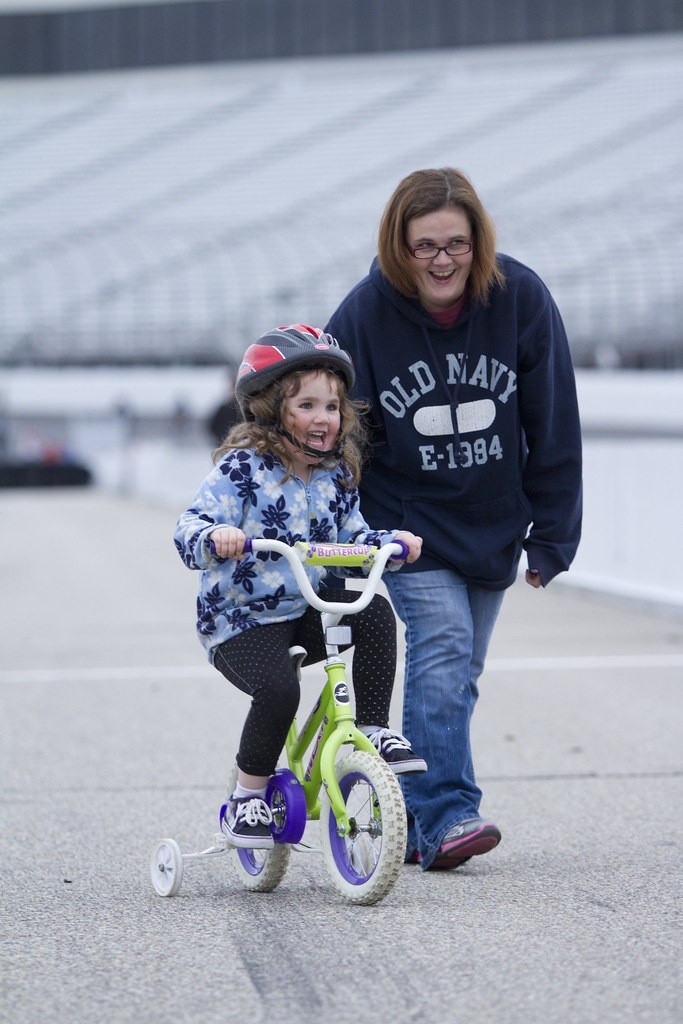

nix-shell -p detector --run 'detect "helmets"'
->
[235,324,355,423]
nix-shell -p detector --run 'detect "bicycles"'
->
[151,536,421,910]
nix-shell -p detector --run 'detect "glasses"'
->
[403,229,475,259]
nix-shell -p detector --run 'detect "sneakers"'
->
[354,727,427,774]
[222,794,274,849]
[431,819,501,871]
[408,847,421,863]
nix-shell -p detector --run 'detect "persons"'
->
[175,324,429,848]
[319,170,583,872]
